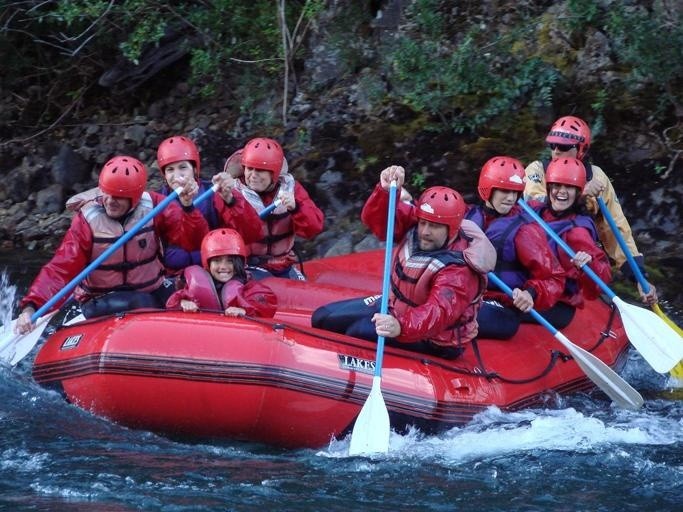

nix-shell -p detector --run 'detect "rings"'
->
[386,325,388,331]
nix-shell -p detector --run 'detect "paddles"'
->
[0,294,76,368]
[486,269,644,409]
[518,192,683,411]
[1,177,191,363]
[349,180,398,457]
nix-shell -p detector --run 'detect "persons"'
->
[524,116,658,306]
[225,138,323,280]
[14,155,206,333]
[399,154,566,339]
[154,136,264,291]
[524,158,612,329]
[312,165,497,359]
[167,227,278,320]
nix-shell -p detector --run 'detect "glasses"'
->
[548,142,577,153]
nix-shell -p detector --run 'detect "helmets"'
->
[200,228,247,270]
[415,186,466,239]
[98,156,147,207]
[240,137,283,184]
[545,115,590,157]
[157,136,201,179]
[545,158,586,196]
[478,156,525,202]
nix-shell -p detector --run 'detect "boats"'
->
[32,247,632,457]
